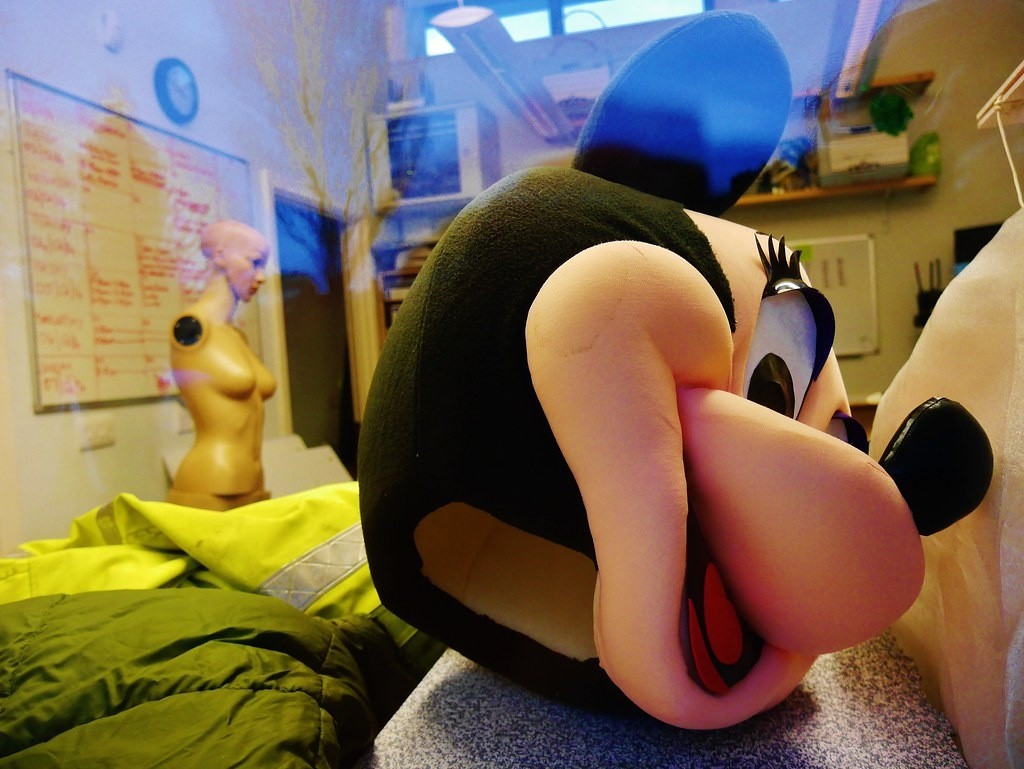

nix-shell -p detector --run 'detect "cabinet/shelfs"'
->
[731,69,937,204]
[371,234,445,345]
[368,100,500,216]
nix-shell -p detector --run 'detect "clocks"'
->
[152,56,198,124]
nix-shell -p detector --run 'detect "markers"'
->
[836,353,862,358]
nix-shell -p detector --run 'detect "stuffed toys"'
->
[356,11,995,735]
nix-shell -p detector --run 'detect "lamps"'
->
[431,1,574,146]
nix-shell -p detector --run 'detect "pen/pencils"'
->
[913,258,943,290]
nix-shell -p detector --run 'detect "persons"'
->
[164,219,278,509]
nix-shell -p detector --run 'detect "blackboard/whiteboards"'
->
[5,69,263,412]
[786,233,881,359]
